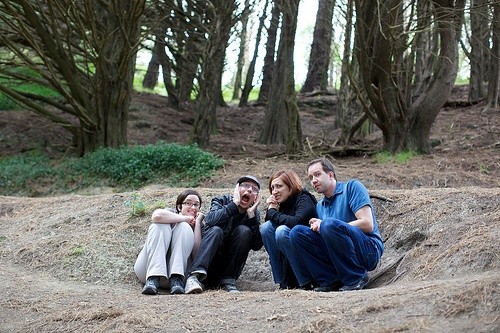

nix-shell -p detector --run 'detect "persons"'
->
[184,175,264,294]
[134,189,205,295]
[259,169,319,291]
[289,158,384,293]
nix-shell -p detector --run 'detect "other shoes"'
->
[314,282,343,292]
[185,276,203,293]
[141,277,160,295]
[169,277,184,294]
[219,283,239,292]
[339,275,370,291]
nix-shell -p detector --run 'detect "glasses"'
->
[240,183,259,194]
[182,202,201,208]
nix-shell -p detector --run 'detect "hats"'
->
[236,175,260,189]
